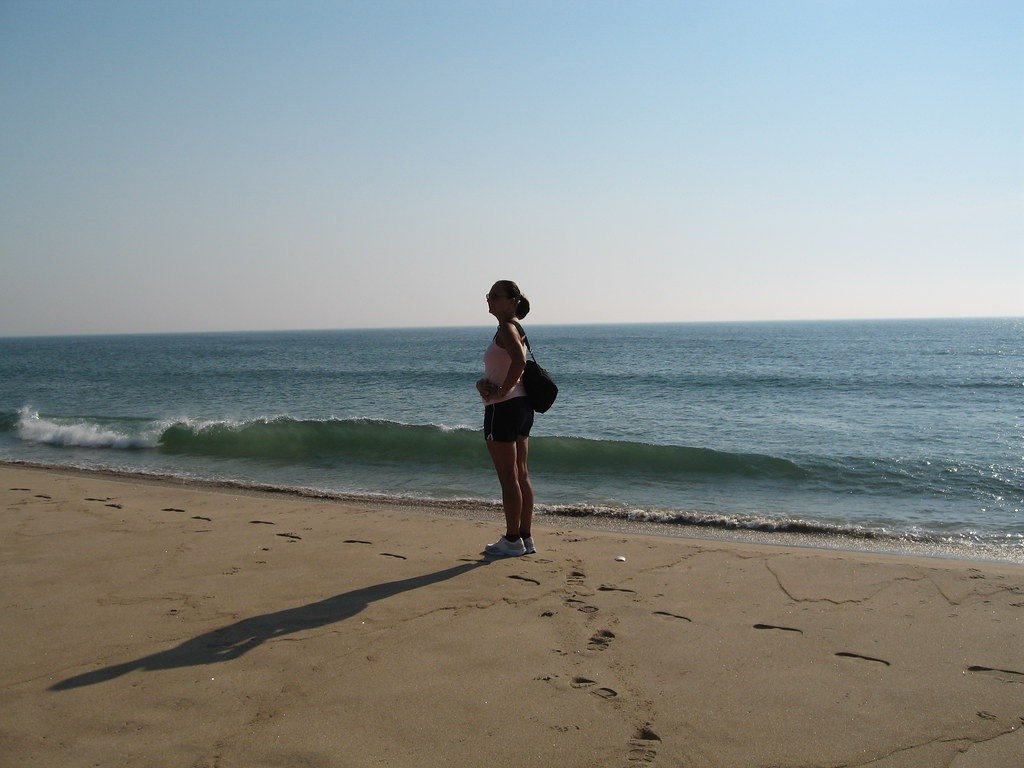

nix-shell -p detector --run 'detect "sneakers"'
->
[485,535,527,556]
[523,537,537,554]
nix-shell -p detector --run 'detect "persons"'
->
[476,280,537,556]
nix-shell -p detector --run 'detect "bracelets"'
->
[497,386,502,399]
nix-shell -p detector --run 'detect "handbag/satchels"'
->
[521,360,559,414]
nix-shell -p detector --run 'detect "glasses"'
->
[486,293,508,300]
